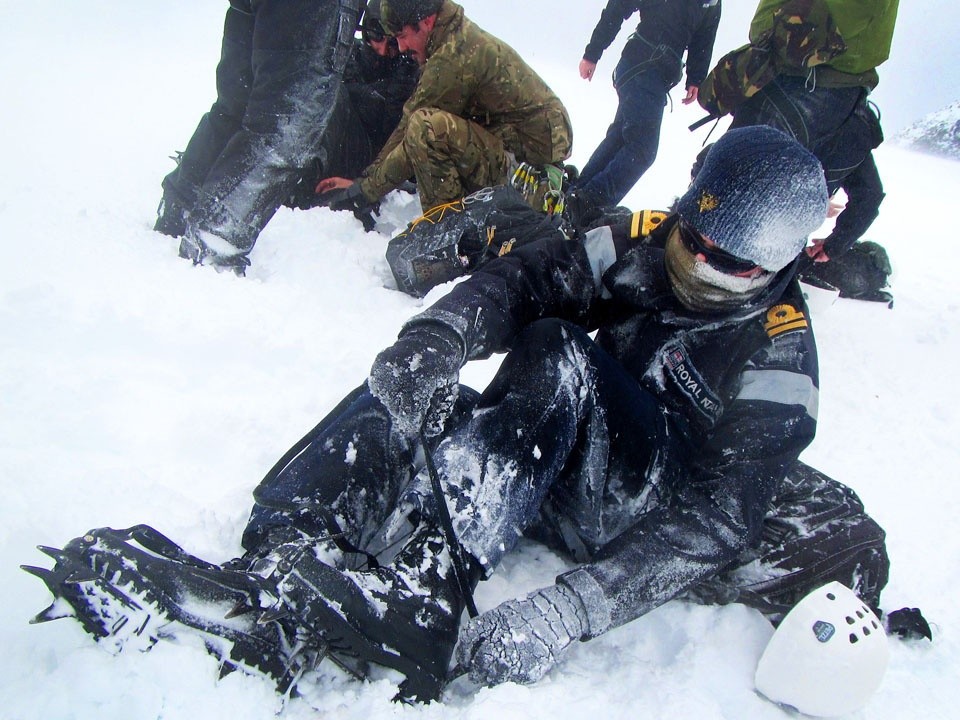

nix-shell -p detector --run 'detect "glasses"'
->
[679,214,762,277]
[366,26,399,49]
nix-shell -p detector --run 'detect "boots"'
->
[278,522,480,700]
[85,501,322,693]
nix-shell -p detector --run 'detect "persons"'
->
[155,0,366,277]
[736,0,900,287]
[280,0,575,227]
[19,127,826,705]
[570,0,723,216]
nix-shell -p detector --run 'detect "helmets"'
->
[756,581,893,720]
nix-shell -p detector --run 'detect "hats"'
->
[381,0,443,34]
[674,122,829,272]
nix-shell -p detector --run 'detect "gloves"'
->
[456,586,586,689]
[324,178,367,210]
[368,324,464,439]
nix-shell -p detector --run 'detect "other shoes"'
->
[181,223,242,281]
[156,187,191,237]
[812,240,864,296]
